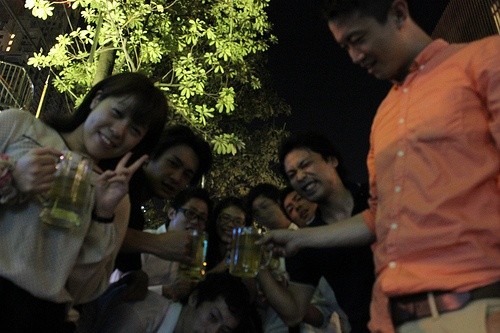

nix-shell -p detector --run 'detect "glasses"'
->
[178,207,208,224]
[220,216,243,226]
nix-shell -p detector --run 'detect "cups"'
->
[176,229,210,283]
[229,227,273,280]
[37,149,95,229]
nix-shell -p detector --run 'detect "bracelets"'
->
[1,154,29,212]
[92,209,115,224]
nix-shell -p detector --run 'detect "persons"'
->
[0,72,168,333]
[118,124,371,333]
[256,1,500,333]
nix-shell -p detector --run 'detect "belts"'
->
[389,280,500,327]
